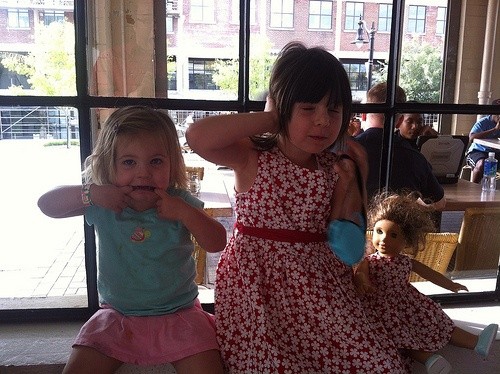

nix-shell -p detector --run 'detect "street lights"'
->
[350,13,377,104]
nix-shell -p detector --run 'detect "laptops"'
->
[415,135,469,184]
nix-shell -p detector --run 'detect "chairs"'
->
[183,166,212,284]
[367,208,500,283]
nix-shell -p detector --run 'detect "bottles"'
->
[482,151,498,193]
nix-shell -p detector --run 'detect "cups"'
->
[188,172,200,192]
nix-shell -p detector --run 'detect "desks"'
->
[196,171,500,234]
[473,138,500,150]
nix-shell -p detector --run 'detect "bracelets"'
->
[81,183,94,206]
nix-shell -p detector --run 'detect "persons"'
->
[465,99,500,183]
[348,83,444,204]
[37,105,226,374]
[354,193,497,374]
[185,42,412,374]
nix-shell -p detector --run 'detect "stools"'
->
[460,166,474,184]
